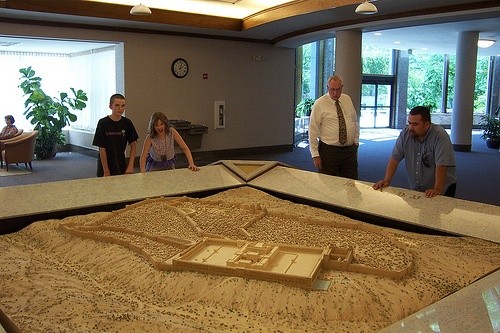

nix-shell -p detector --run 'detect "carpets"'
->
[0,161,32,176]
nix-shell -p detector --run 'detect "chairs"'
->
[0,129,39,172]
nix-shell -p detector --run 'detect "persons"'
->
[139,111,200,172]
[373,106,457,198]
[92,94,139,177]
[0,115,18,140]
[308,75,359,180]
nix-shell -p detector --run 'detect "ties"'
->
[335,100,348,145]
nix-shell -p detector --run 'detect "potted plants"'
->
[478,111,500,149]
[17,66,88,159]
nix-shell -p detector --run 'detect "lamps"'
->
[130,3,152,15]
[355,0,378,14]
[478,39,495,48]
[253,55,264,63]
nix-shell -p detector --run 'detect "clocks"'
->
[172,58,188,78]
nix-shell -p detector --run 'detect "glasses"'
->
[421,155,430,168]
